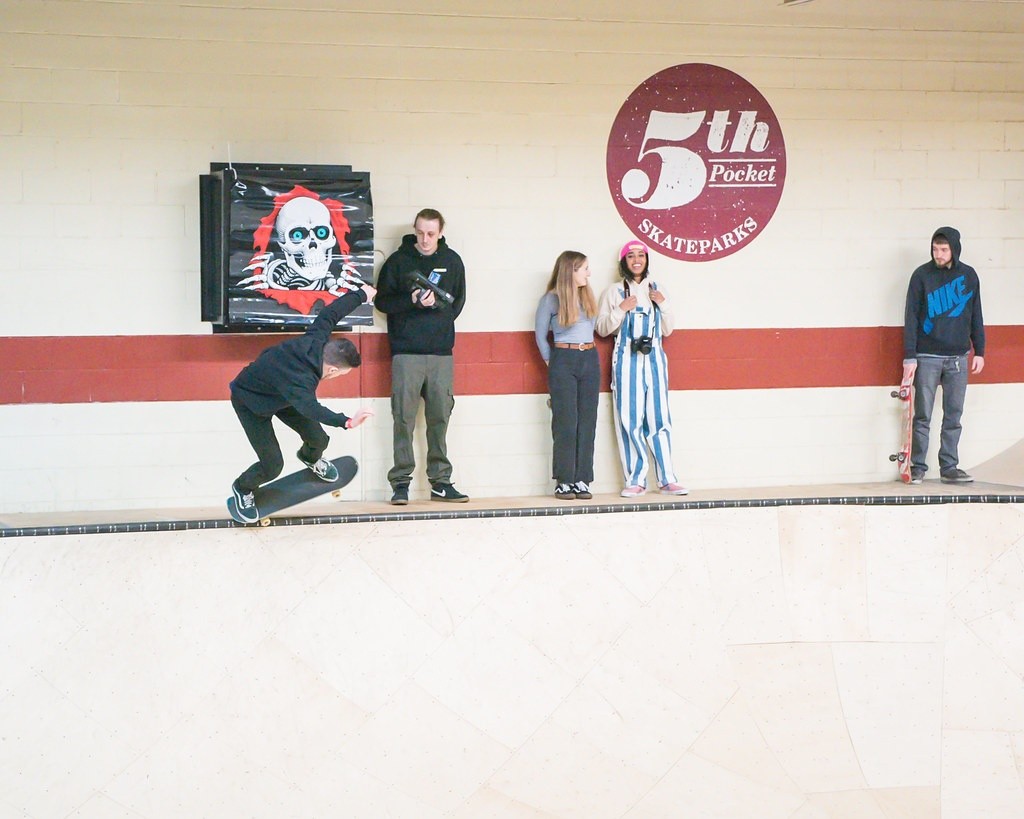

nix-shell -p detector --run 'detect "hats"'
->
[619,240,649,262]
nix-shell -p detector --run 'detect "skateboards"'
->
[888,373,914,484]
[225,455,359,527]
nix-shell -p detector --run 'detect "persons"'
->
[598,240,688,499]
[374,209,471,506]
[897,225,987,485]
[226,283,378,526]
[532,250,599,501]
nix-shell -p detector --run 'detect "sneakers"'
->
[390,482,409,505]
[909,466,925,484]
[659,482,688,495]
[621,485,645,497]
[574,480,592,499]
[940,469,974,482]
[231,482,259,523]
[296,450,339,483]
[554,483,575,500]
[430,483,470,504]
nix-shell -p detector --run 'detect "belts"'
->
[554,341,597,352]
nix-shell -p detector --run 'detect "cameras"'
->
[631,335,652,356]
